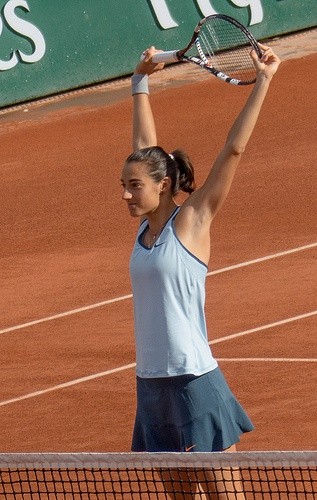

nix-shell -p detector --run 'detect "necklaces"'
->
[146,231,158,249]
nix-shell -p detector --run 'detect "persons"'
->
[121,44,280,500]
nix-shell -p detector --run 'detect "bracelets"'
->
[131,74,150,96]
